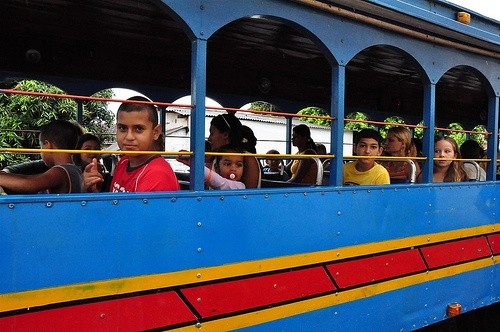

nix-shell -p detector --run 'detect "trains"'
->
[0,0,500,332]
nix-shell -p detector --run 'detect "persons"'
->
[181,112,331,190]
[0,95,178,195]
[343,126,500,186]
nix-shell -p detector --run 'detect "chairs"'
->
[97,153,481,193]
